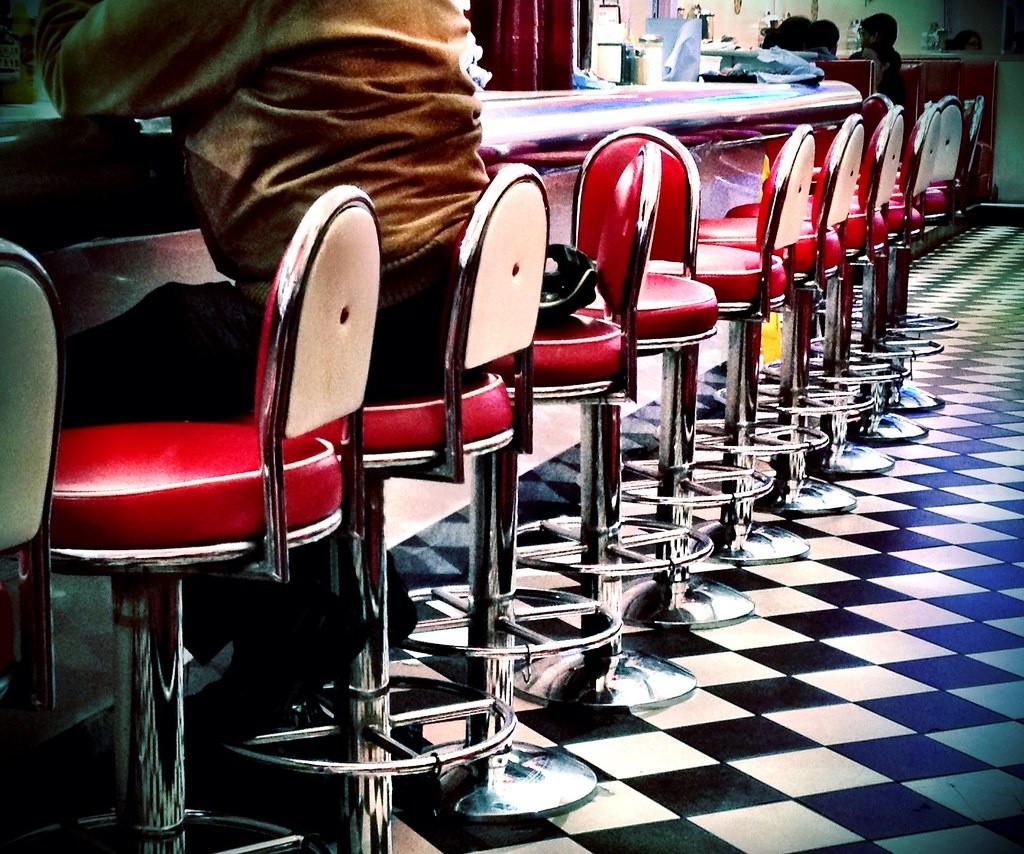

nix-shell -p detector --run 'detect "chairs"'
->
[848,95,966,446]
[0,237,60,854]
[702,111,869,564]
[805,102,944,479]
[508,123,718,713]
[46,182,385,854]
[224,162,552,854]
[618,125,818,633]
[755,101,910,520]
[886,94,987,413]
[810,91,895,190]
[410,140,665,825]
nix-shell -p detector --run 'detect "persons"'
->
[949,29,983,52]
[761,16,840,60]
[20,1,490,734]
[847,12,906,122]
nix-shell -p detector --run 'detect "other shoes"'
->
[385,594,417,645]
[186,593,365,729]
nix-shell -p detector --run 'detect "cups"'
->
[597,42,623,82]
[636,34,662,85]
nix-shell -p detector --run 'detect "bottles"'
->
[847,17,865,51]
[0,0,37,105]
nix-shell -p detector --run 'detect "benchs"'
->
[818,59,1002,262]
